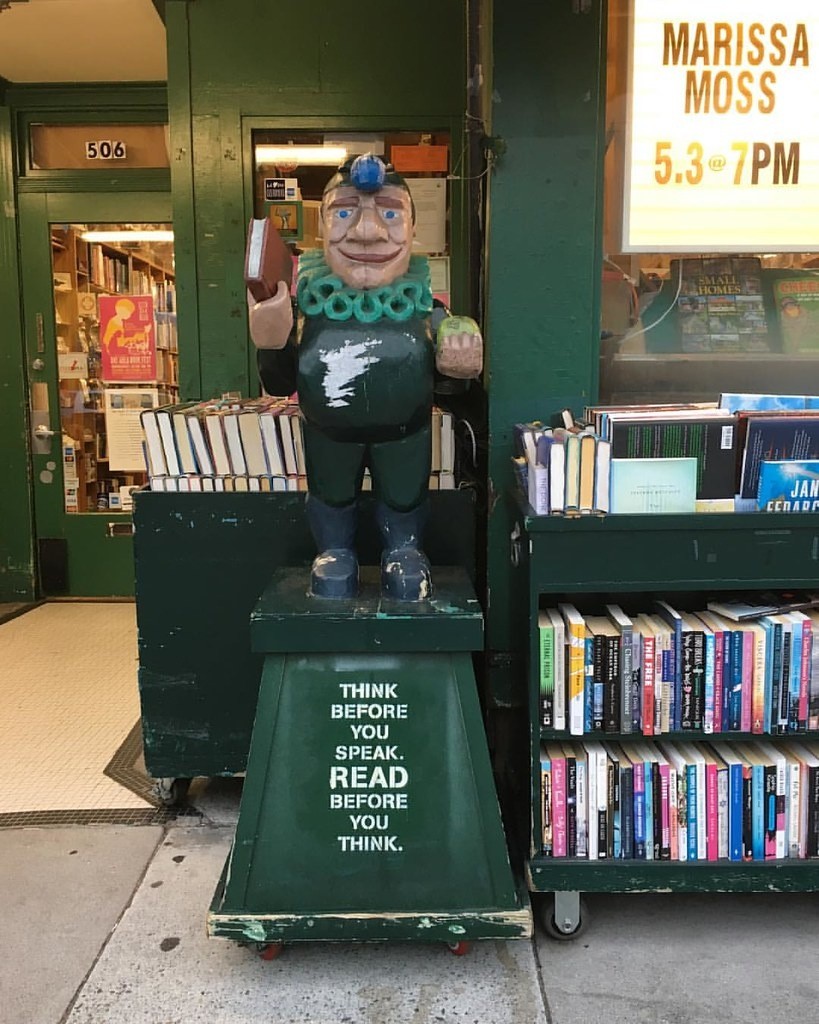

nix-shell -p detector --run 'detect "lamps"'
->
[253,141,348,168]
[81,230,175,242]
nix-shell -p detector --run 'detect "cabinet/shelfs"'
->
[48,222,179,514]
[507,479,819,941]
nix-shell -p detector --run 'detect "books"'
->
[139,392,460,496]
[665,257,773,355]
[773,274,819,356]
[513,393,817,514]
[53,225,176,384]
[539,594,814,862]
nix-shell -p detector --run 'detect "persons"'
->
[244,152,481,598]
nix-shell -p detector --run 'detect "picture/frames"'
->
[264,200,304,241]
